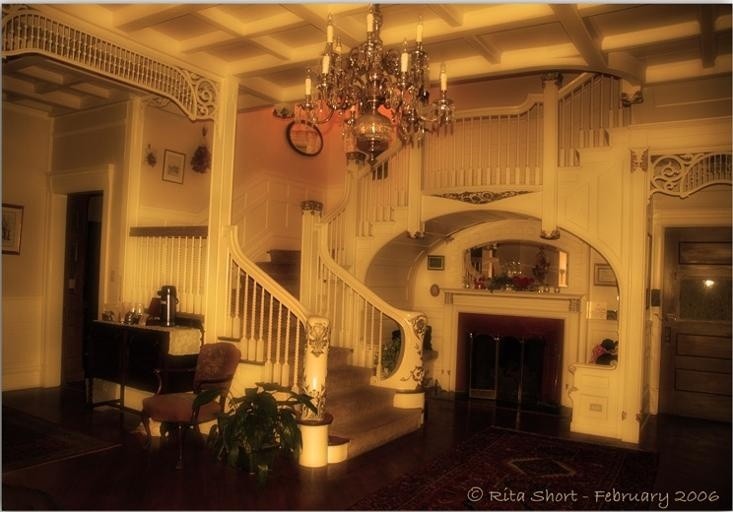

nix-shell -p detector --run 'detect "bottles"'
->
[506,256,522,280]
[156,285,177,327]
[463,263,484,290]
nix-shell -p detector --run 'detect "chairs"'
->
[140,342,241,470]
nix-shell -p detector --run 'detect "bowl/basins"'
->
[509,276,534,291]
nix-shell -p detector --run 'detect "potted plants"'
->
[206,381,317,483]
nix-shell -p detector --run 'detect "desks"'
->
[84,321,202,421]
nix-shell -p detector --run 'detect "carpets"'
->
[2,404,122,475]
[343,425,658,511]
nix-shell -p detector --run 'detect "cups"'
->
[119,302,144,325]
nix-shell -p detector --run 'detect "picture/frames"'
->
[161,148,186,185]
[427,255,445,270]
[1,203,24,256]
[678,241,732,265]
[593,263,617,287]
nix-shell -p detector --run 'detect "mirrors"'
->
[463,239,570,288]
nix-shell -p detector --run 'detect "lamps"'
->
[294,3,455,166]
